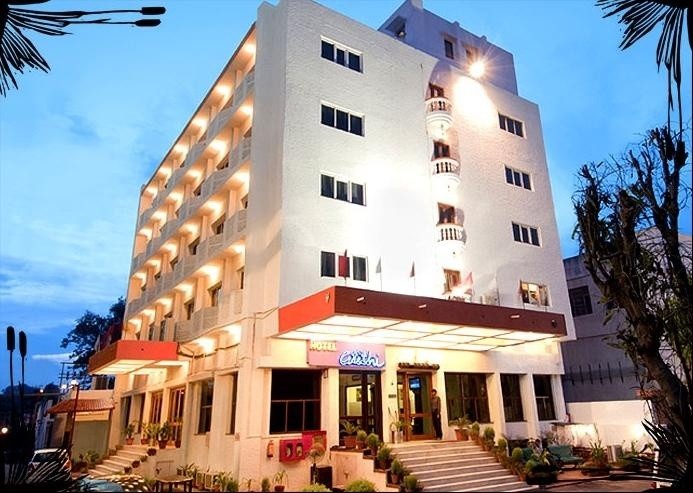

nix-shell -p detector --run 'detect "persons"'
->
[429,389,443,440]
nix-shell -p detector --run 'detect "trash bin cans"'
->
[607,446,624,464]
[310,465,333,490]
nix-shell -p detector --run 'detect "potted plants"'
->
[273,470,288,492]
[339,406,423,492]
[123,417,182,449]
[453,414,536,480]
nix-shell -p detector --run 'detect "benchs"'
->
[519,445,584,472]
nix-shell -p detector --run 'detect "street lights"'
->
[70,379,80,447]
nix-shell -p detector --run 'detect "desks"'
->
[154,474,194,493]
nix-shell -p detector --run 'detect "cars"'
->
[28,447,71,483]
